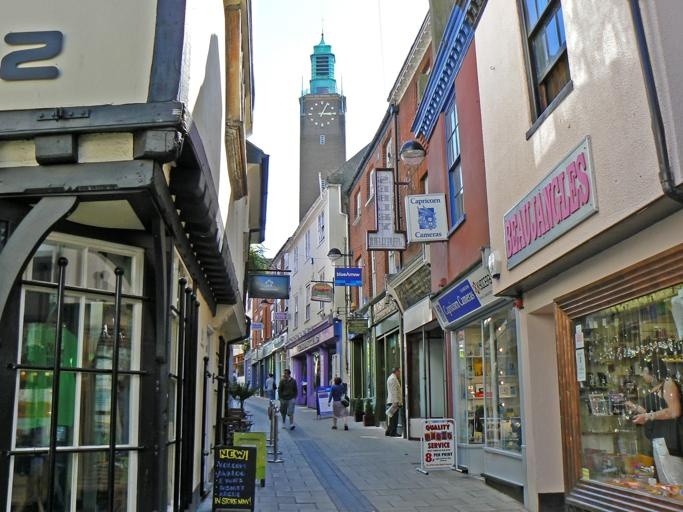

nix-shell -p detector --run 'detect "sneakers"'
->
[290,425,296,430]
[344,426,348,430]
[281,424,285,428]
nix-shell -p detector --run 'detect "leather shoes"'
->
[331,426,337,429]
[391,433,401,437]
[385,431,392,435]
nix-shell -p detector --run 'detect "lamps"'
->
[399,142,430,167]
[326,248,353,259]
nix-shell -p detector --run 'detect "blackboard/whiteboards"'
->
[213,445,257,509]
[423,422,454,469]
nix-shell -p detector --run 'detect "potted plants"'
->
[354,395,375,426]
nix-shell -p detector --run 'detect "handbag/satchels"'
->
[341,384,349,407]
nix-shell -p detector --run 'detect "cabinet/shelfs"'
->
[464,300,683,481]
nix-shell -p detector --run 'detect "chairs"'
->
[221,408,255,446]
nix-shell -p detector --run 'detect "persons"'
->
[265,374,274,400]
[327,377,348,430]
[627,355,683,489]
[384,366,403,436]
[232,369,237,384]
[277,369,297,431]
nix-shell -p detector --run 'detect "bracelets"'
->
[647,413,654,420]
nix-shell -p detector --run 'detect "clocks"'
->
[308,99,337,128]
